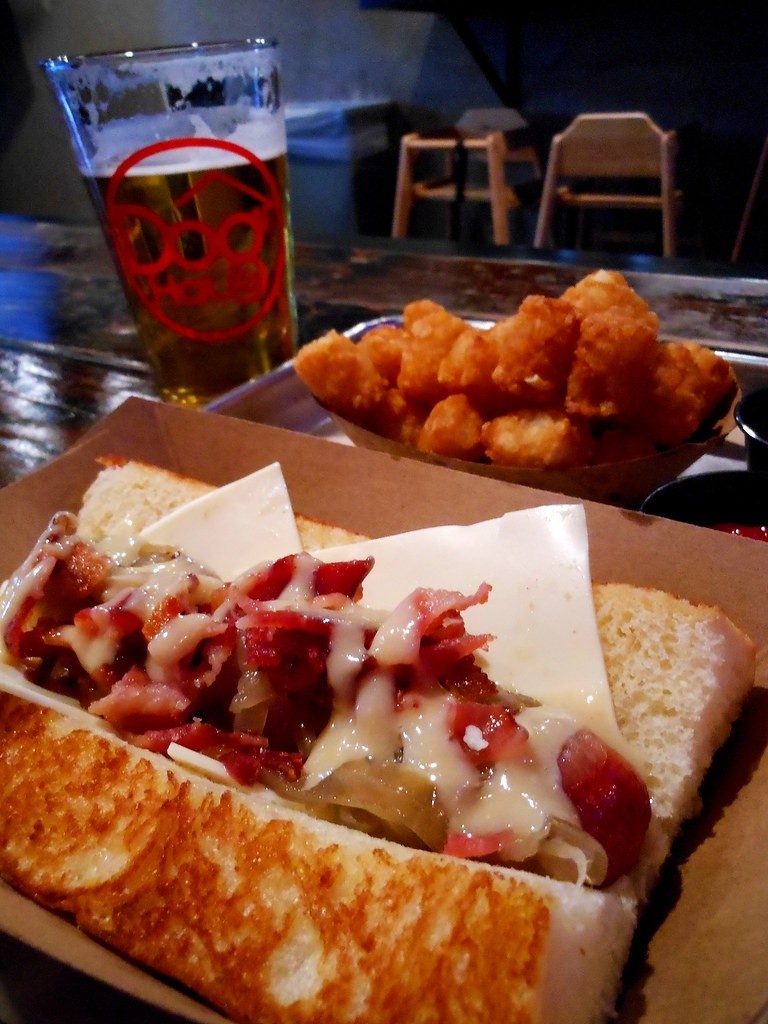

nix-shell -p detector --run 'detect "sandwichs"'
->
[0,455,760,1022]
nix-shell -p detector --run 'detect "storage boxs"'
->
[2,397,768,1024]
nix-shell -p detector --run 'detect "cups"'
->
[38,34,295,408]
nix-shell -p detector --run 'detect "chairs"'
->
[390,108,538,244]
[533,111,681,258]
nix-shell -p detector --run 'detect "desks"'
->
[0,217,768,522]
